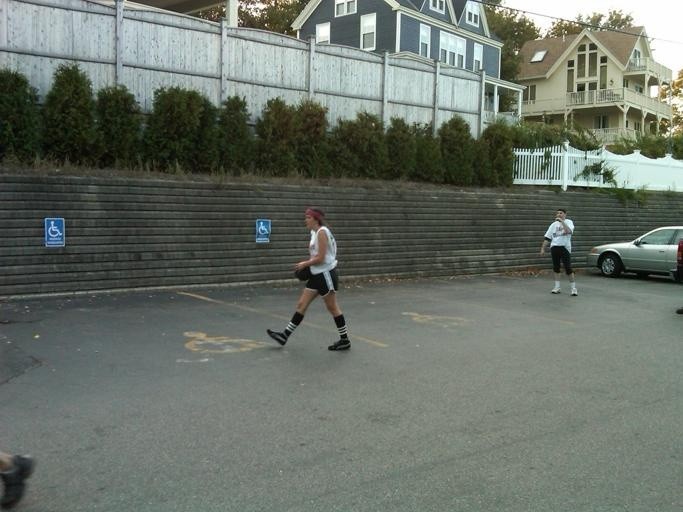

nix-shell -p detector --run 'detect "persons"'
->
[266,206,351,351]
[540,210,578,297]
[1,450,33,511]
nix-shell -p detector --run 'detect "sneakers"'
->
[329,339,350,350]
[571,287,578,296]
[552,288,561,294]
[267,329,288,345]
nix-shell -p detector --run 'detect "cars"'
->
[586,224,683,278]
